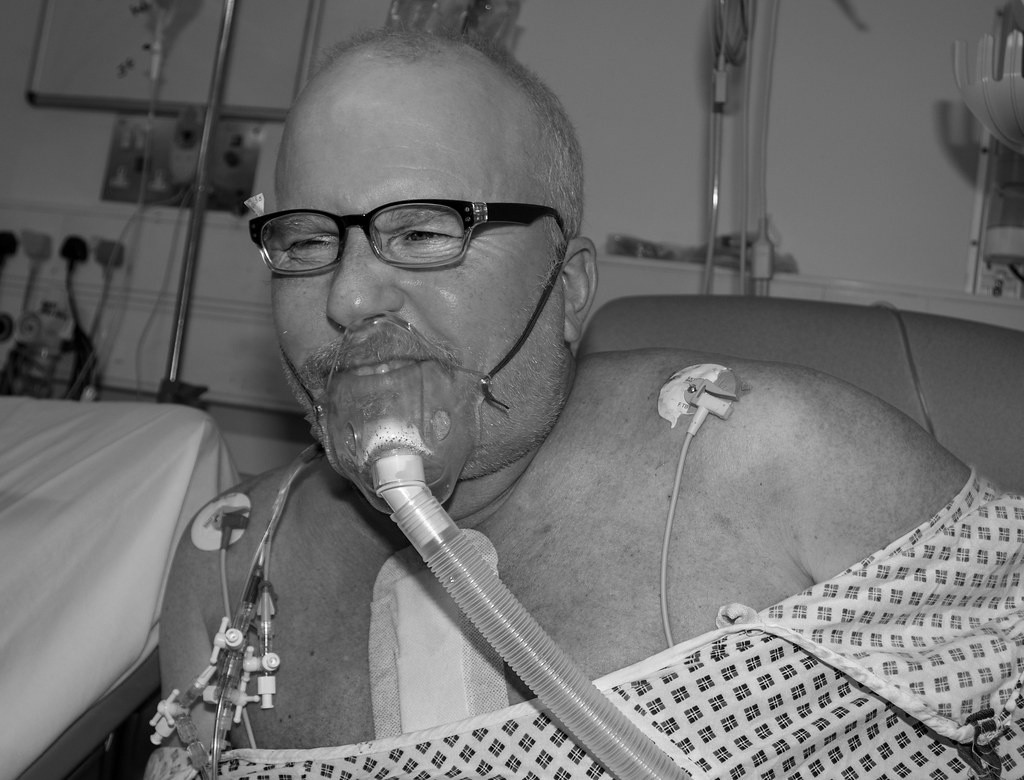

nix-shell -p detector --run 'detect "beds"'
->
[1,397,244,780]
[579,293,1023,503]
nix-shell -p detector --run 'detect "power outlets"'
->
[0,207,134,287]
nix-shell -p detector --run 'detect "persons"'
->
[159,31,1024,780]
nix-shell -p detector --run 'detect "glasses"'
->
[249,197,569,278]
